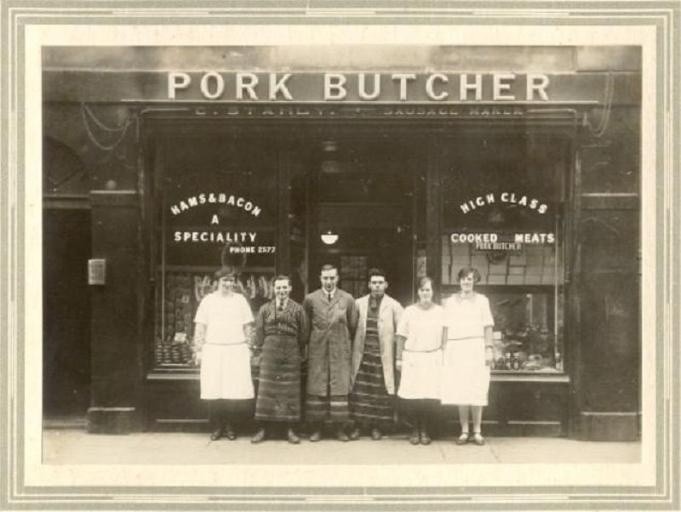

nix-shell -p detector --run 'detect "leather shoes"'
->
[455,431,486,446]
[288,431,300,445]
[250,428,269,443]
[211,425,222,441]
[226,430,236,440]
[411,433,432,445]
[310,429,322,443]
[334,427,382,441]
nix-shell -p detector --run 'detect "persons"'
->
[190,267,257,440]
[302,265,361,442]
[393,276,448,445]
[344,268,404,440]
[444,266,497,446]
[252,275,309,444]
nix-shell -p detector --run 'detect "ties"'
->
[278,300,284,311]
[328,294,331,301]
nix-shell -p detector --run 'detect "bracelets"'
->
[485,344,493,350]
